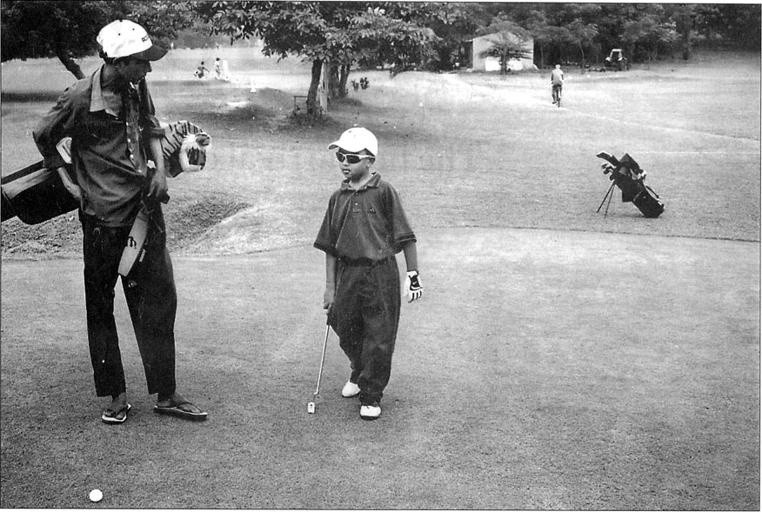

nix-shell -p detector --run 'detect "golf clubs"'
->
[307,308,330,414]
[597,153,618,215]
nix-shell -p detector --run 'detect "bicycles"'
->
[551,81,562,108]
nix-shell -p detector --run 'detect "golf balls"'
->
[88,489,104,502]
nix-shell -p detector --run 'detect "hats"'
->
[96,19,169,62]
[327,125,378,160]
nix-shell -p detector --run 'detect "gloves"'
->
[400,270,424,304]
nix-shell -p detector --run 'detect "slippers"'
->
[101,401,132,425]
[153,402,209,420]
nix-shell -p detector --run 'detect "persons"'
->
[550,64,565,104]
[196,61,210,80]
[312,126,424,420]
[32,16,211,425]
[213,58,221,79]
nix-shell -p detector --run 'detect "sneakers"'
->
[359,405,381,420]
[340,378,360,399]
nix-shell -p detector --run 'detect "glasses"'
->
[334,151,376,165]
